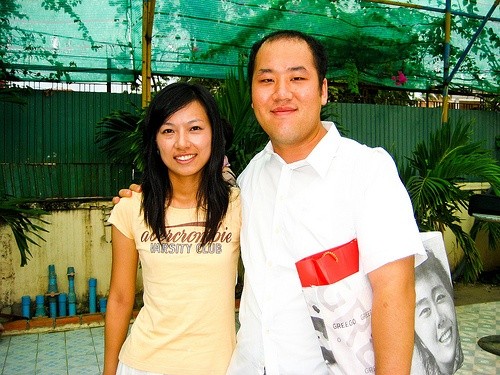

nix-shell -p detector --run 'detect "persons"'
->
[101,81,241,375]
[411,250,465,375]
[109,29,430,375]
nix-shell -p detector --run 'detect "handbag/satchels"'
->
[408,230,465,375]
